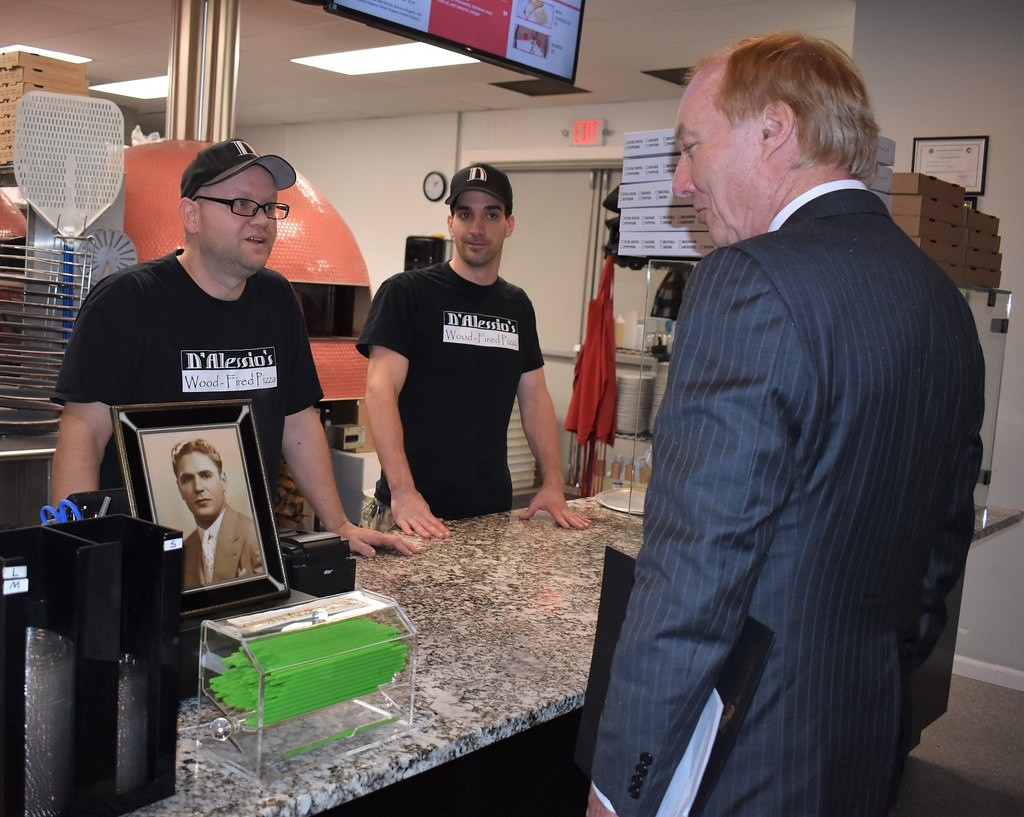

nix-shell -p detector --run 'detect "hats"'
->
[444,162,513,205]
[180,138,297,198]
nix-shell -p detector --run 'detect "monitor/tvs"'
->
[294,0,586,85]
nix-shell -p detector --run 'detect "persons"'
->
[50,138,422,557]
[172,439,264,591]
[356,163,592,539]
[582,34,985,817]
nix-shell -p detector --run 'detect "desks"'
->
[123,486,645,817]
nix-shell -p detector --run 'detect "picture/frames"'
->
[108,397,291,622]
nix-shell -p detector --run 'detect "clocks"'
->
[423,171,446,202]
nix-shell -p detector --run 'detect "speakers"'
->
[403,236,446,273]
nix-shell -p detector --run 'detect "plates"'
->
[615,361,670,434]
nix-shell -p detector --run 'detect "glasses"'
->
[192,195,290,220]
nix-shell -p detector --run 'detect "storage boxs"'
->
[0,51,92,166]
[615,126,1004,289]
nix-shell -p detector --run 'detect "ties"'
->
[201,531,215,587]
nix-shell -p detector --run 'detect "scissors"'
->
[39,499,82,525]
